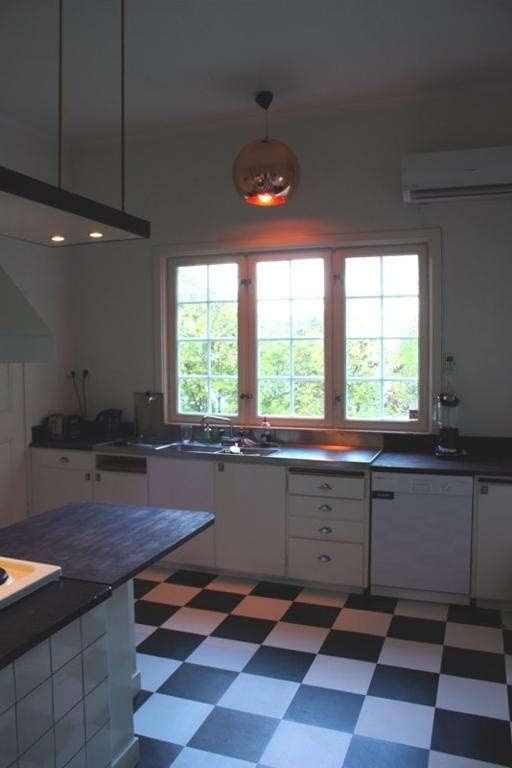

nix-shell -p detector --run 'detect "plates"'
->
[285,470,366,589]
[32,451,149,514]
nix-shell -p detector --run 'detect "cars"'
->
[48,412,65,441]
[179,424,194,442]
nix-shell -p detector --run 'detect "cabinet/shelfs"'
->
[260,416,272,444]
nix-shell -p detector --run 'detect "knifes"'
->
[398,144,512,205]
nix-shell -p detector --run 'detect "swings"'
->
[432,392,460,459]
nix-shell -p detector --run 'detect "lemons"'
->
[95,408,124,438]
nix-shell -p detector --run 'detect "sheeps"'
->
[201,415,234,438]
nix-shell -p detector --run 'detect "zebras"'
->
[371,471,474,595]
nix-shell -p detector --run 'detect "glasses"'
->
[228,88,301,208]
